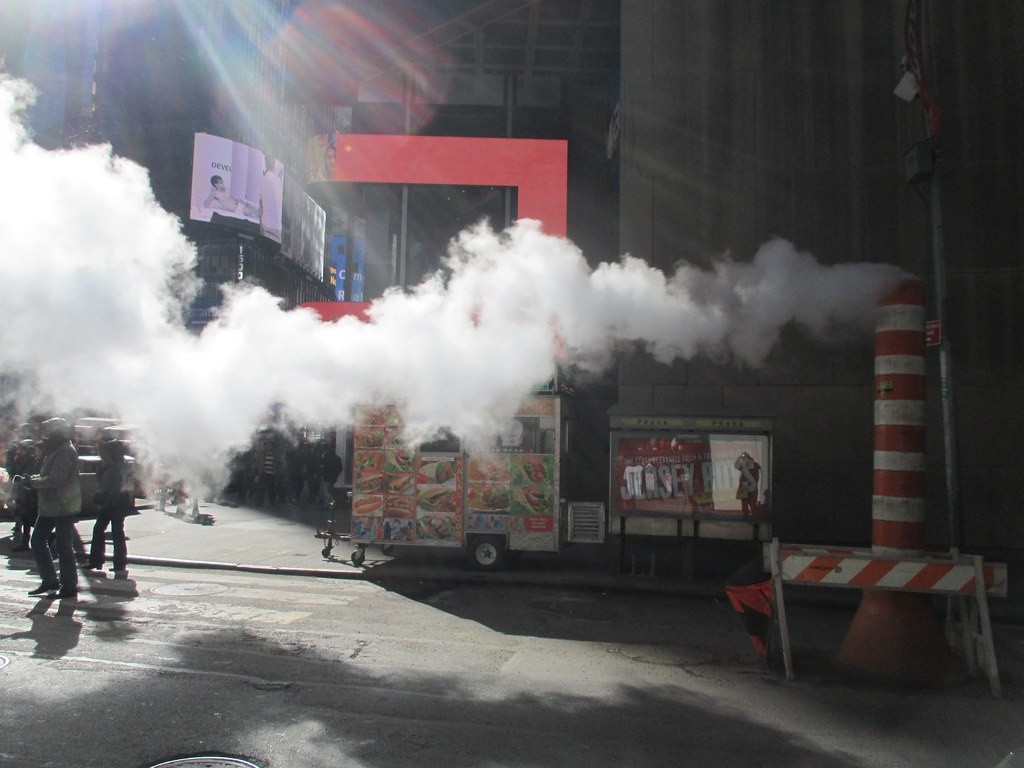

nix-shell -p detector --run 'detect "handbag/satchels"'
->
[82,493,135,517]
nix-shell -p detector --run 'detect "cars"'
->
[0,407,155,516]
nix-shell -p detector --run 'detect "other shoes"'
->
[110,566,126,572]
[82,562,102,570]
[29,579,60,597]
[48,589,78,600]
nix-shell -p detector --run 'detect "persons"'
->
[732,451,761,517]
[324,144,336,180]
[205,175,263,217]
[85,440,129,571]
[257,154,283,240]
[233,437,342,509]
[6,414,88,600]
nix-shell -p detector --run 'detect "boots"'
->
[9,531,30,551]
